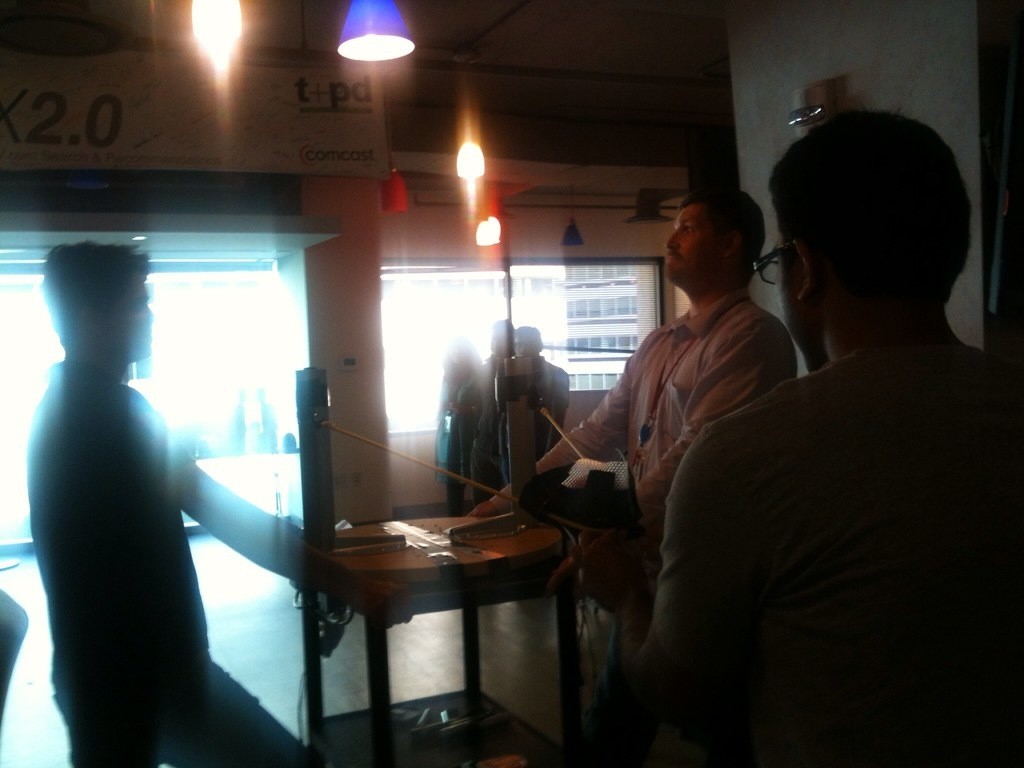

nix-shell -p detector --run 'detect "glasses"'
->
[753,241,800,285]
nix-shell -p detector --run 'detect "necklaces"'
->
[635,339,696,461]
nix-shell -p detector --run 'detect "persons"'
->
[640,107,1023,767]
[465,188,798,768]
[26,240,415,768]
[434,319,570,518]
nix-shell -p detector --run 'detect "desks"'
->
[318,517,581,768]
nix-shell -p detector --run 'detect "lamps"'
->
[622,187,674,224]
[337,0,415,62]
[560,217,584,246]
